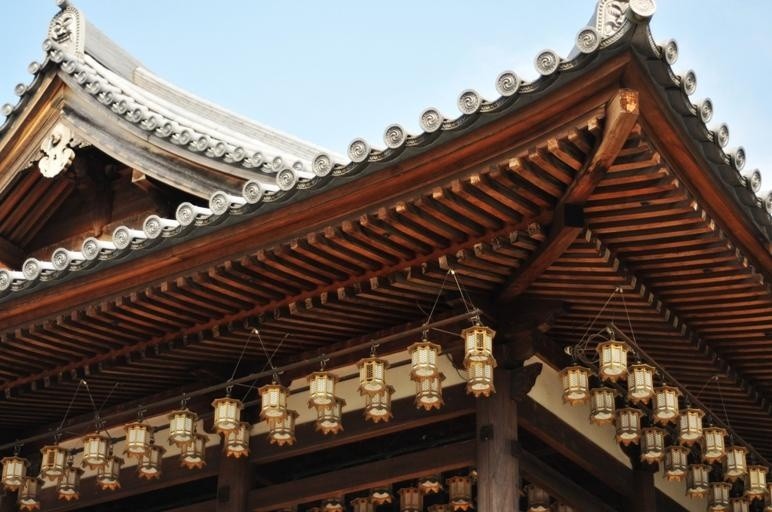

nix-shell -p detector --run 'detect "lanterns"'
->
[2,324,497,512]
[558,341,771,512]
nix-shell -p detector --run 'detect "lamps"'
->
[96,441,125,491]
[136,433,167,480]
[467,365,496,397]
[700,415,728,465]
[54,454,85,502]
[662,440,691,482]
[705,471,733,511]
[587,383,616,426]
[314,396,346,436]
[311,464,580,512]
[208,386,244,433]
[733,495,750,512]
[180,425,210,469]
[267,408,300,447]
[80,423,113,470]
[614,401,647,448]
[558,361,590,408]
[356,346,389,399]
[306,362,337,410]
[121,412,158,458]
[640,421,665,464]
[460,318,499,370]
[2,447,34,492]
[651,373,684,428]
[684,459,712,500]
[39,436,71,481]
[415,372,446,411]
[707,435,749,485]
[596,328,637,383]
[743,465,769,502]
[15,478,43,510]
[258,376,291,424]
[223,421,251,460]
[407,332,441,383]
[364,385,396,423]
[626,352,660,406]
[166,401,198,448]
[676,395,717,447]
